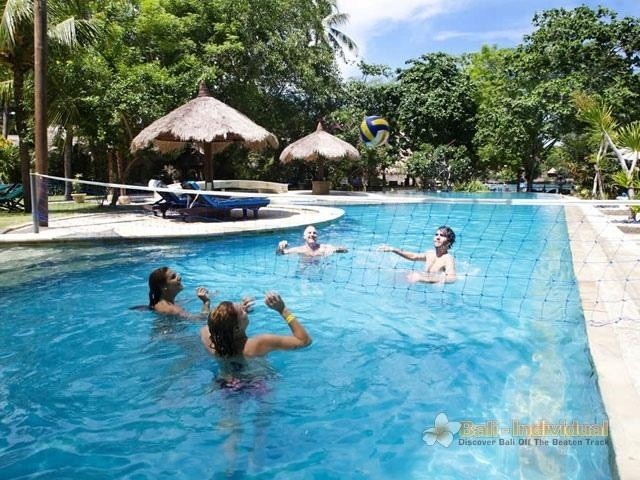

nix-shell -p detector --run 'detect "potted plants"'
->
[71,177,87,203]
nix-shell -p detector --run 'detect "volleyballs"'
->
[359,116,390,145]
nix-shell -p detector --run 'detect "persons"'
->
[147,169,168,187]
[375,224,458,287]
[274,224,349,276]
[196,289,314,383]
[128,265,211,323]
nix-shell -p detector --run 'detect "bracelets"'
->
[286,314,297,324]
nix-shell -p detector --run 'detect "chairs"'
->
[0,181,26,213]
[152,179,271,222]
